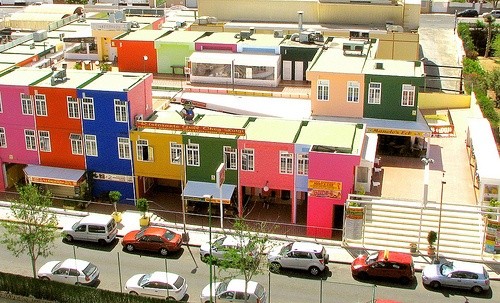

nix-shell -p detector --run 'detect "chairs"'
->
[372,181,381,190]
[51,69,66,82]
[373,167,381,176]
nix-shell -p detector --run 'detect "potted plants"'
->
[353,183,365,206]
[410,243,417,252]
[484,198,500,222]
[109,191,122,222]
[61,192,111,211]
[136,198,149,226]
[426,231,437,255]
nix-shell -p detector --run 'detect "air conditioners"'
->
[30,42,35,49]
[135,113,144,127]
[59,33,65,41]
[274,30,283,38]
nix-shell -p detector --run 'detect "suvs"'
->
[200,279,267,303]
[124,271,188,301]
[200,235,260,268]
[266,241,330,276]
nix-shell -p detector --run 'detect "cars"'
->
[350,249,415,283]
[121,227,183,256]
[422,260,490,295]
[489,10,500,18]
[37,258,99,286]
[457,9,478,17]
[61,214,118,246]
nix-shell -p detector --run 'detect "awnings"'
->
[180,180,238,205]
[22,165,87,188]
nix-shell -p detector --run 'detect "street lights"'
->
[437,180,447,258]
[203,194,212,303]
